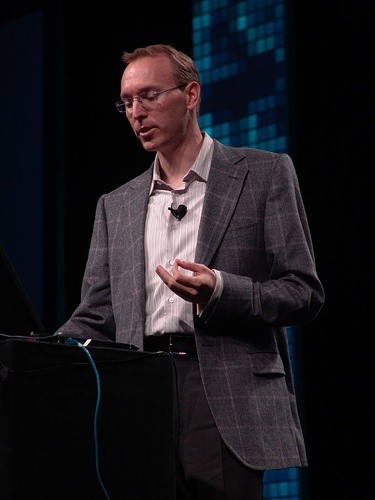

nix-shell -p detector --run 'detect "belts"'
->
[144,334,197,357]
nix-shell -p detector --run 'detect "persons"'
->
[53,44,326,500]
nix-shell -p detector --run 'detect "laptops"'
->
[0,242,140,351]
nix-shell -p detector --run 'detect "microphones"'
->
[168,205,187,221]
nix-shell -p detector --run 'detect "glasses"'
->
[115,83,189,113]
